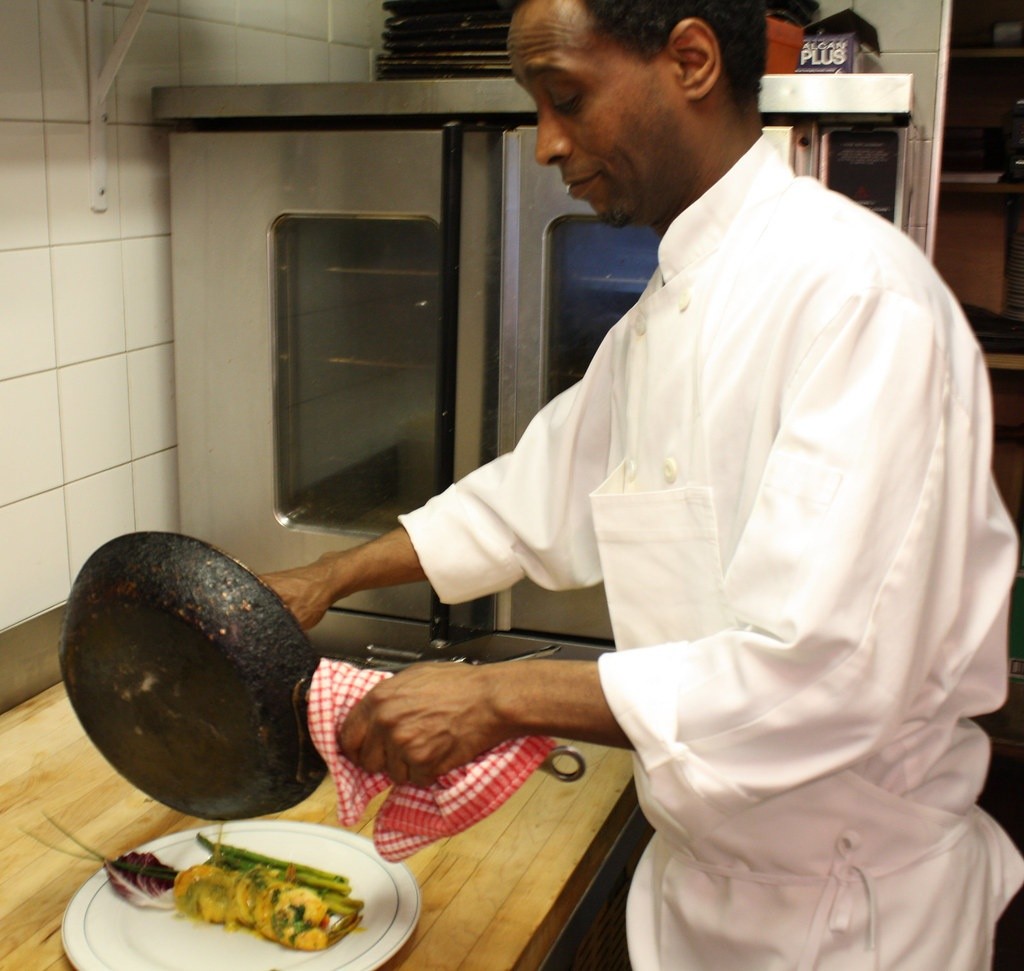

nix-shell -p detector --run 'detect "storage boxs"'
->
[795,32,881,74]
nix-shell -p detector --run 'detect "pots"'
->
[58,531,587,822]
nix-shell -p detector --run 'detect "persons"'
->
[252,1,1024,971]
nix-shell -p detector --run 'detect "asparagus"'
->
[197,832,365,917]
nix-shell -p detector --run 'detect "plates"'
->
[61,820,421,971]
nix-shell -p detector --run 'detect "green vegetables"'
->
[99,851,180,910]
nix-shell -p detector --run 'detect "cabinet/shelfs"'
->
[940,47,1024,371]
